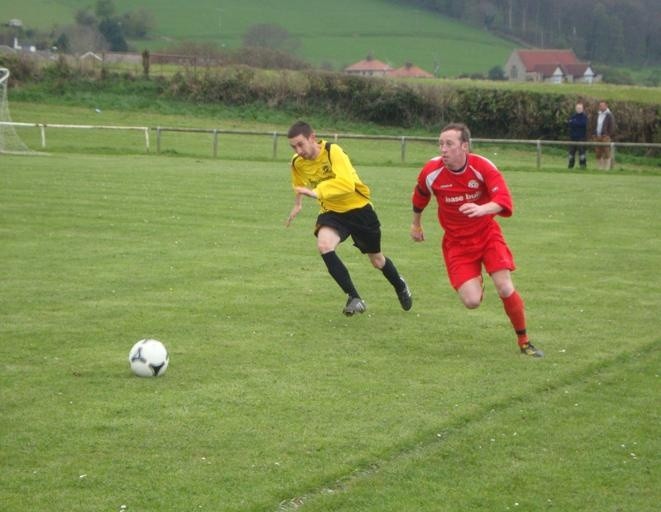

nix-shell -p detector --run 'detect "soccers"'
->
[129,339,169,377]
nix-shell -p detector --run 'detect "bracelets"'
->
[411,224,423,232]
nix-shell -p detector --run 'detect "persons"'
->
[284,120,413,316]
[409,122,545,358]
[568,102,589,168]
[594,100,618,170]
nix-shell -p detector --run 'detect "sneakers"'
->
[342,296,367,317]
[519,341,545,358]
[394,275,413,311]
